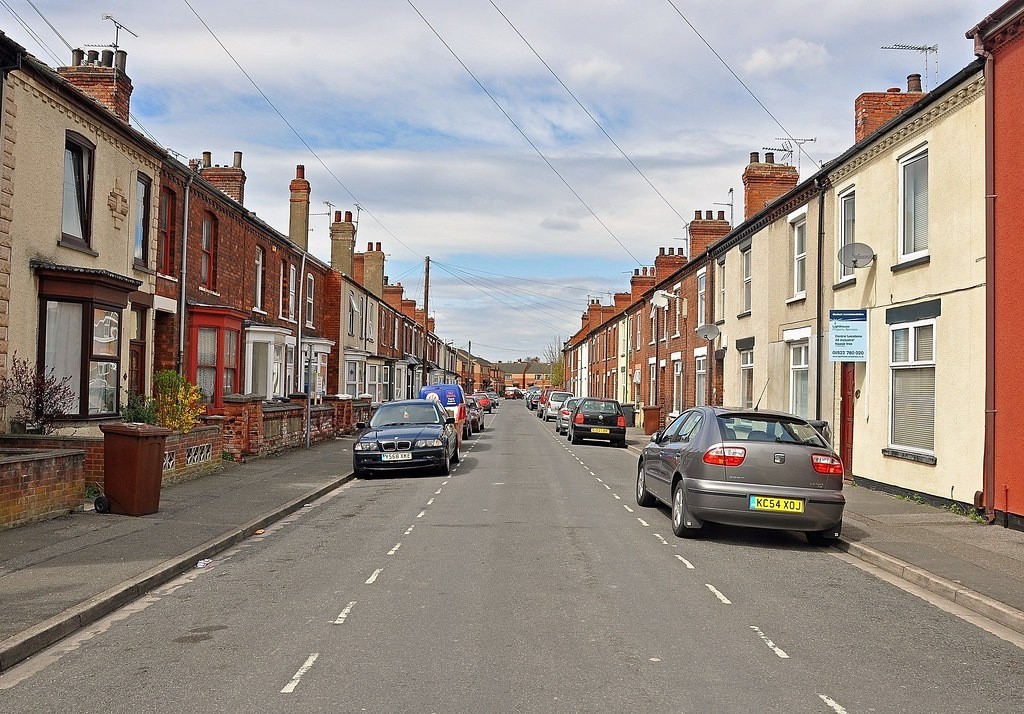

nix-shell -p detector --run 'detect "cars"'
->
[567,397,627,448]
[472,385,574,421]
[465,395,485,433]
[352,399,460,479]
[636,405,846,547]
[554,396,587,436]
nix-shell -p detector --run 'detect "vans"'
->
[417,383,475,440]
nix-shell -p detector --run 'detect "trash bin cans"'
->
[621,404,635,426]
[642,405,662,435]
[94,422,170,516]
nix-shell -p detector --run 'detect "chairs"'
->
[725,428,737,439]
[748,431,766,440]
